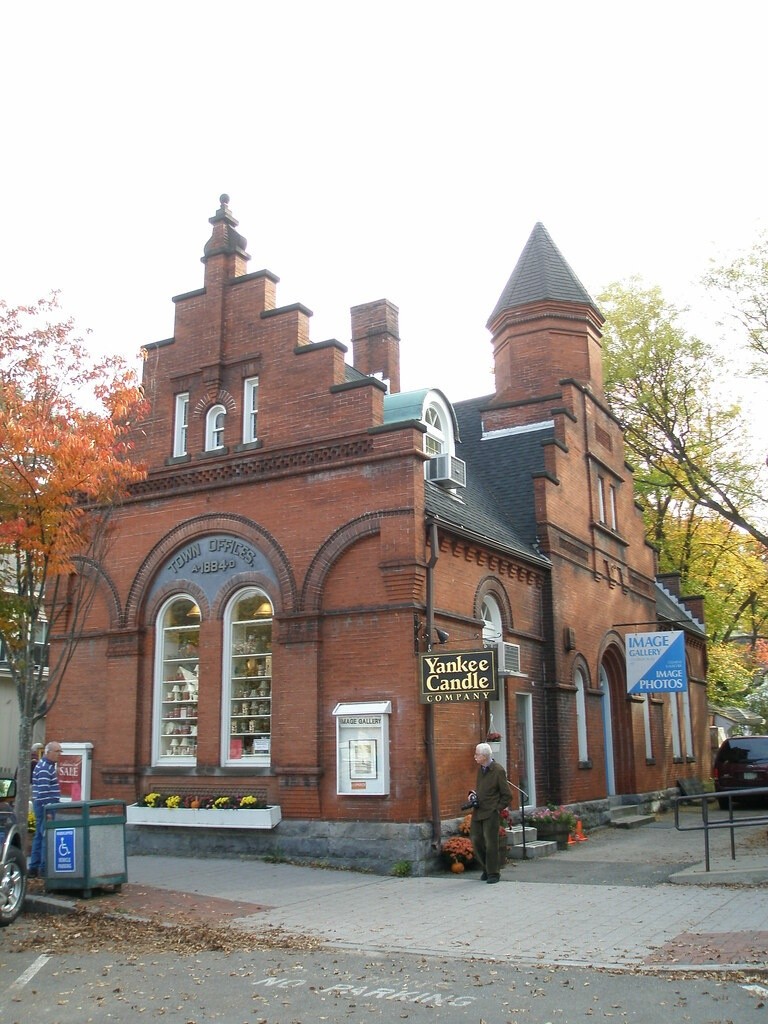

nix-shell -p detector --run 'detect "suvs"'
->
[712,734,768,810]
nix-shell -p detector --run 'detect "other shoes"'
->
[27,869,40,878]
[481,871,487,880]
[486,876,499,884]
[36,871,44,879]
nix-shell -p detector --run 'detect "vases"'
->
[440,852,464,866]
[529,821,569,849]
[126,802,282,830]
[498,837,507,870]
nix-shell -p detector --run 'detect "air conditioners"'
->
[484,641,520,674]
[426,453,465,488]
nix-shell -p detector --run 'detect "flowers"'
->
[458,808,512,836]
[134,792,268,810]
[443,836,474,860]
[526,801,578,838]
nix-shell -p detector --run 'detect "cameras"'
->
[461,795,479,811]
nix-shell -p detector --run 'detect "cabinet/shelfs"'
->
[225,617,273,762]
[158,625,200,762]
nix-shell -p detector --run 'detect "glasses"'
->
[55,750,64,753]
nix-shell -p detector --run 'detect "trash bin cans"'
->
[41,799,128,898]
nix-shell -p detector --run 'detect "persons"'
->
[28,742,62,880]
[14,742,45,785]
[468,743,513,884]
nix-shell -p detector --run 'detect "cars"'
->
[0,778,28,928]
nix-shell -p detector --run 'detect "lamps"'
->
[563,641,576,658]
[414,627,449,643]
